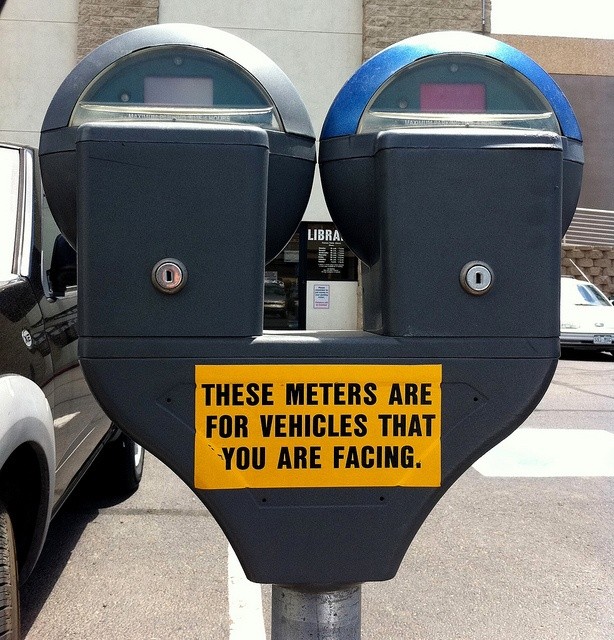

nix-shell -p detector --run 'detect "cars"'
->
[0,142,142,640]
[556,266,614,356]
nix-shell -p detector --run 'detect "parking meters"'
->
[39,21,586,640]
